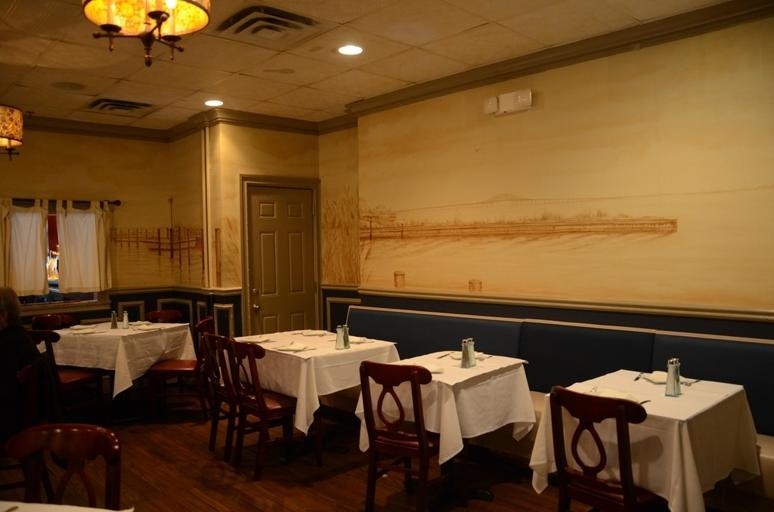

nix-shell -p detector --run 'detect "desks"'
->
[527,365,765,512]
[1,495,139,512]
[219,328,408,475]
[354,345,537,499]
[36,318,197,423]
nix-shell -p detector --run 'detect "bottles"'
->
[334,324,351,350]
[665,358,683,396]
[110,310,128,329]
[460,336,476,369]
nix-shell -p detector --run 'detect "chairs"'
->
[143,315,219,426]
[144,308,191,323]
[545,382,668,511]
[201,329,274,456]
[356,362,461,511]
[219,337,323,479]
[30,315,75,334]
[0,418,123,512]
[1,328,107,428]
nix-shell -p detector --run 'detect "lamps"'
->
[1,103,26,159]
[74,0,216,67]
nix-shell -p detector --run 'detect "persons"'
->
[0,285,54,444]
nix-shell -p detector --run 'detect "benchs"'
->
[332,303,772,512]
